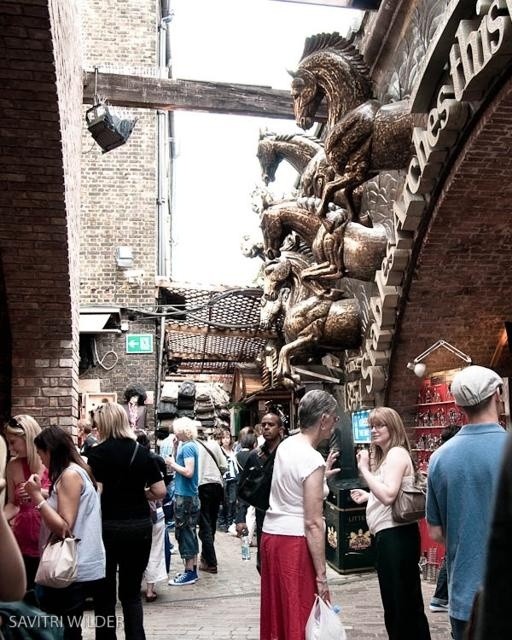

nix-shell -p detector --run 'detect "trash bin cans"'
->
[322,477,377,576]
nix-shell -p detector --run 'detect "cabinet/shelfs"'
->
[409,399,466,477]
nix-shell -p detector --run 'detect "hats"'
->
[450,365,503,406]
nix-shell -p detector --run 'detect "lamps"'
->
[80,66,139,156]
[404,337,478,379]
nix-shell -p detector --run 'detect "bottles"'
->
[239,527,252,562]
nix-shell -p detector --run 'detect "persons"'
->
[425,364,509,639]
[0,392,341,640]
[350,406,431,640]
[428,424,464,612]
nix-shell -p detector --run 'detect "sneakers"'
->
[168,565,217,585]
[430,602,449,612]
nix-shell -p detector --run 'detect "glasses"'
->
[6,416,24,430]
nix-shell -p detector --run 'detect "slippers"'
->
[146,592,156,601]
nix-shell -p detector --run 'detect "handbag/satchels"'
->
[34,521,81,589]
[238,437,285,510]
[392,486,426,523]
